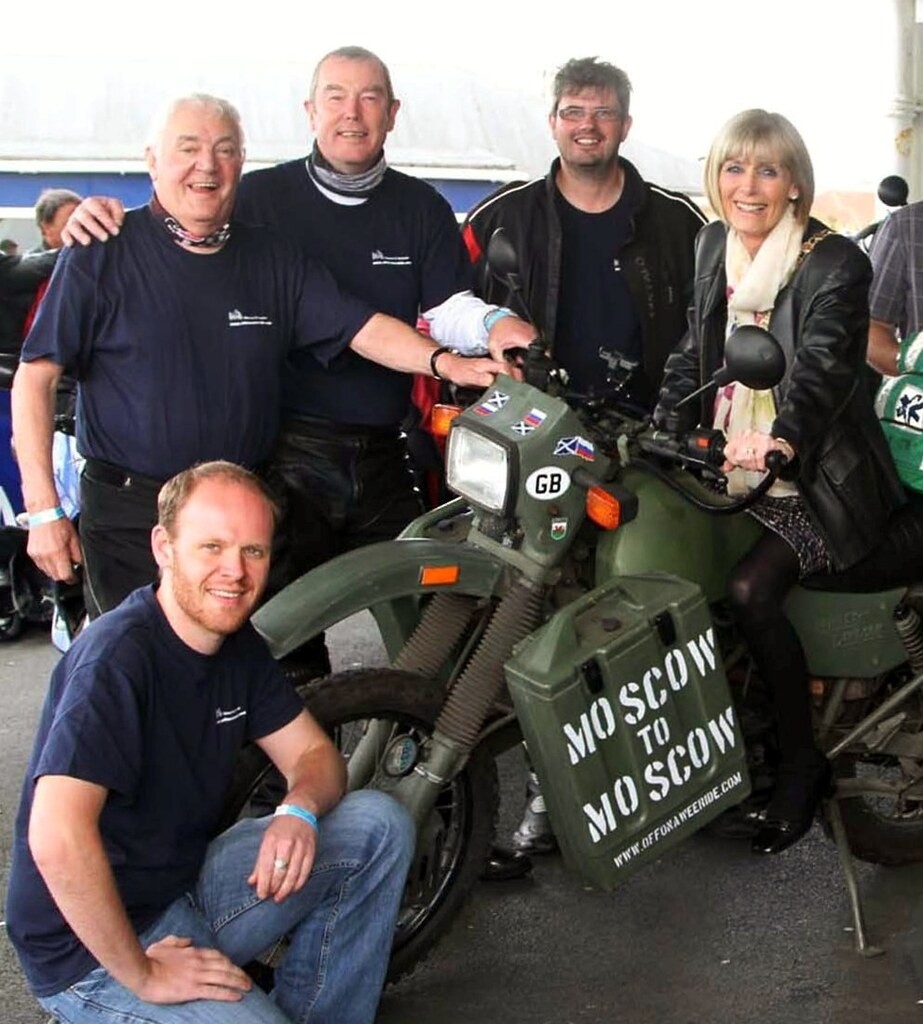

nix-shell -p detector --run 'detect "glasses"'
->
[556,104,619,123]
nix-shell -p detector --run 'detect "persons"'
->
[3,91,522,622]
[0,247,59,354]
[653,109,897,859]
[60,48,549,822]
[6,461,415,1024]
[27,190,84,254]
[471,56,711,854]
[863,201,923,380]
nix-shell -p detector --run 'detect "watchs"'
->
[430,346,461,382]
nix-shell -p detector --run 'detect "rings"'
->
[274,861,288,869]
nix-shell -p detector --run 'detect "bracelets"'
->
[273,804,317,828]
[485,308,519,330]
[25,506,65,526]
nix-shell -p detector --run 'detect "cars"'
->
[1,159,538,656]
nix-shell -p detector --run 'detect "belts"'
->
[81,458,138,488]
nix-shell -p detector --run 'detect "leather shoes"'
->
[478,846,532,880]
[750,759,838,854]
[252,766,289,810]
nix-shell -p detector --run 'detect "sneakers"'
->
[511,777,558,856]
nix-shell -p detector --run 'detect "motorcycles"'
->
[212,227,923,997]
